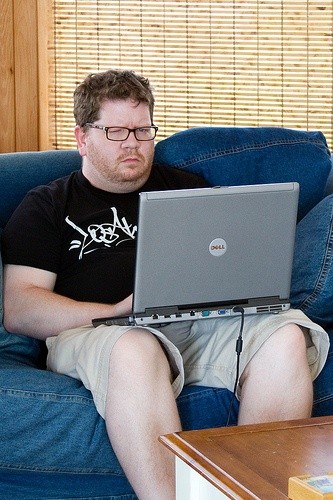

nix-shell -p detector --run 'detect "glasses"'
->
[86,122,158,141]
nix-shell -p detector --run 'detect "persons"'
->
[1,69,333,500]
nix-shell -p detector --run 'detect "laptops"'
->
[90,182,302,328]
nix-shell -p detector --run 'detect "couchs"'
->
[0,126,333,500]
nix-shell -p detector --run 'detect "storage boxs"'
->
[285,472,333,499]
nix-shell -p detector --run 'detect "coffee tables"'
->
[158,416,333,499]
